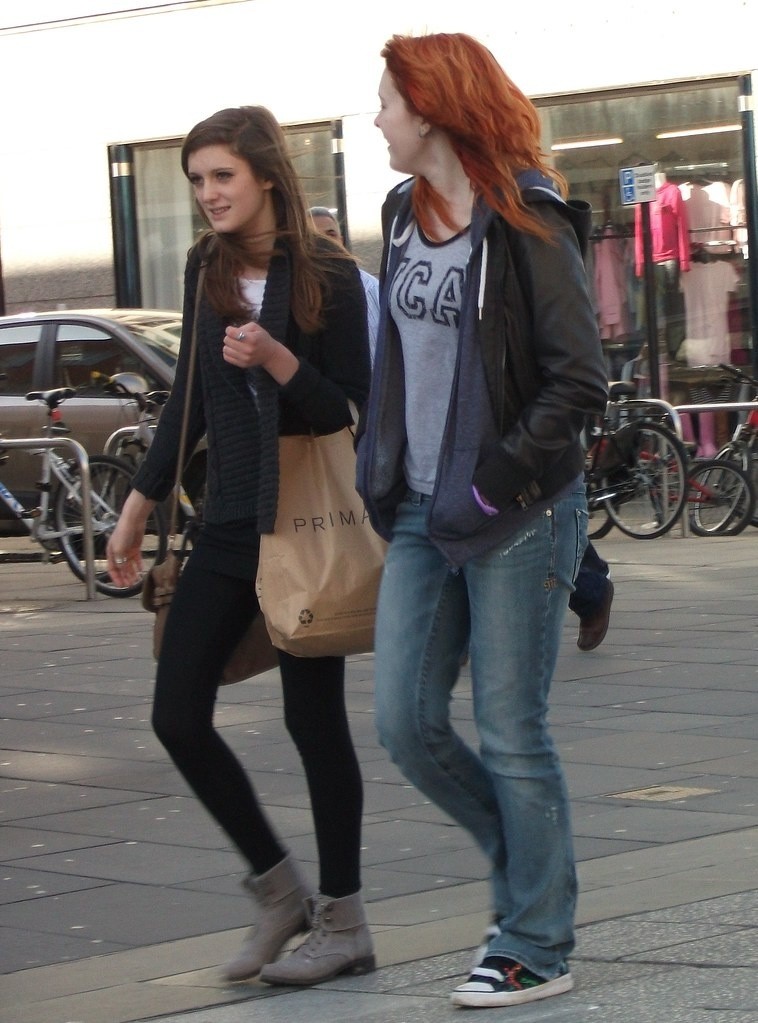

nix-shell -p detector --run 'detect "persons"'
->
[105,103,380,984]
[303,205,615,650]
[352,32,612,1008]
[634,172,693,355]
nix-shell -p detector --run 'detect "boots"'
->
[226,850,312,981]
[260,887,376,985]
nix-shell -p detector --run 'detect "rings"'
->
[238,332,245,341]
[116,558,128,564]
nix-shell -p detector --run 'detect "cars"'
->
[0,310,207,539]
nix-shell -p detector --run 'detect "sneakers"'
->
[450,914,572,1006]
[577,578,614,650]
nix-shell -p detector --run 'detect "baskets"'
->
[688,376,741,411]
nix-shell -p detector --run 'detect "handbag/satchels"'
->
[142,550,279,686]
[255,400,390,658]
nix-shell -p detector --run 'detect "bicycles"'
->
[583,382,755,540]
[92,380,199,585]
[693,363,758,536]
[0,374,166,599]
